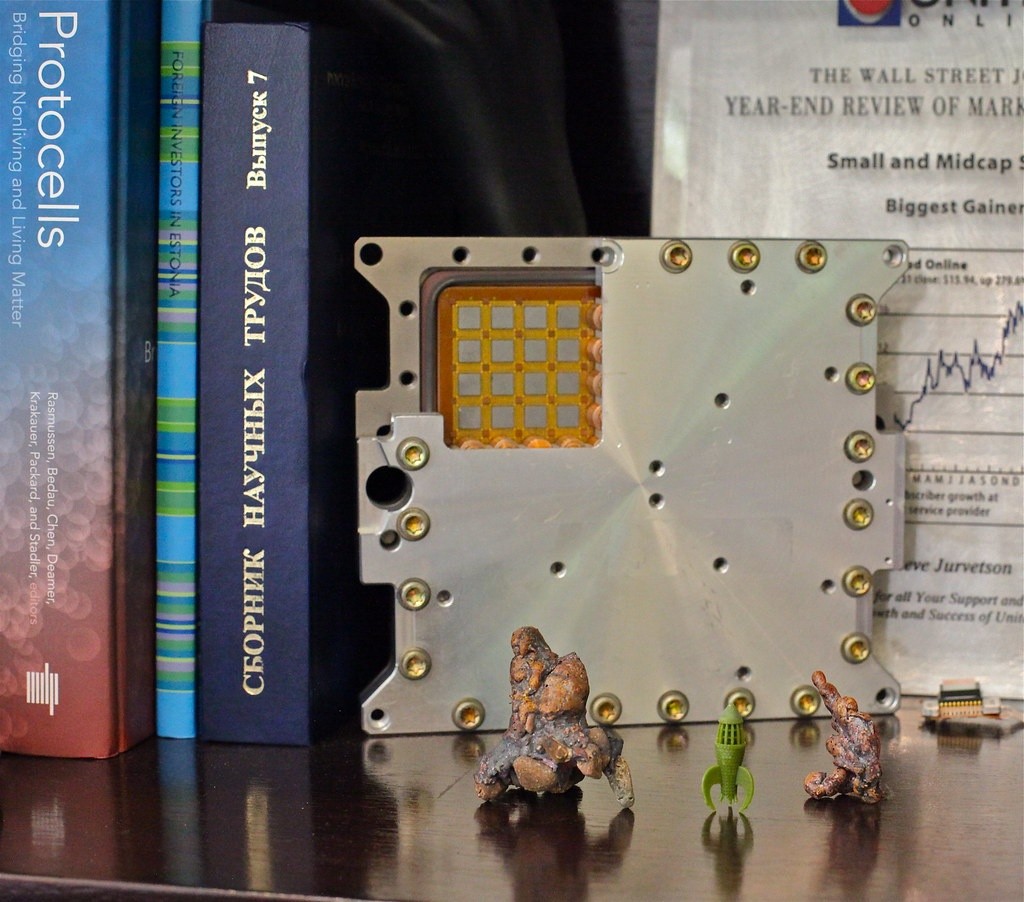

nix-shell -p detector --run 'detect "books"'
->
[1,0,348,761]
